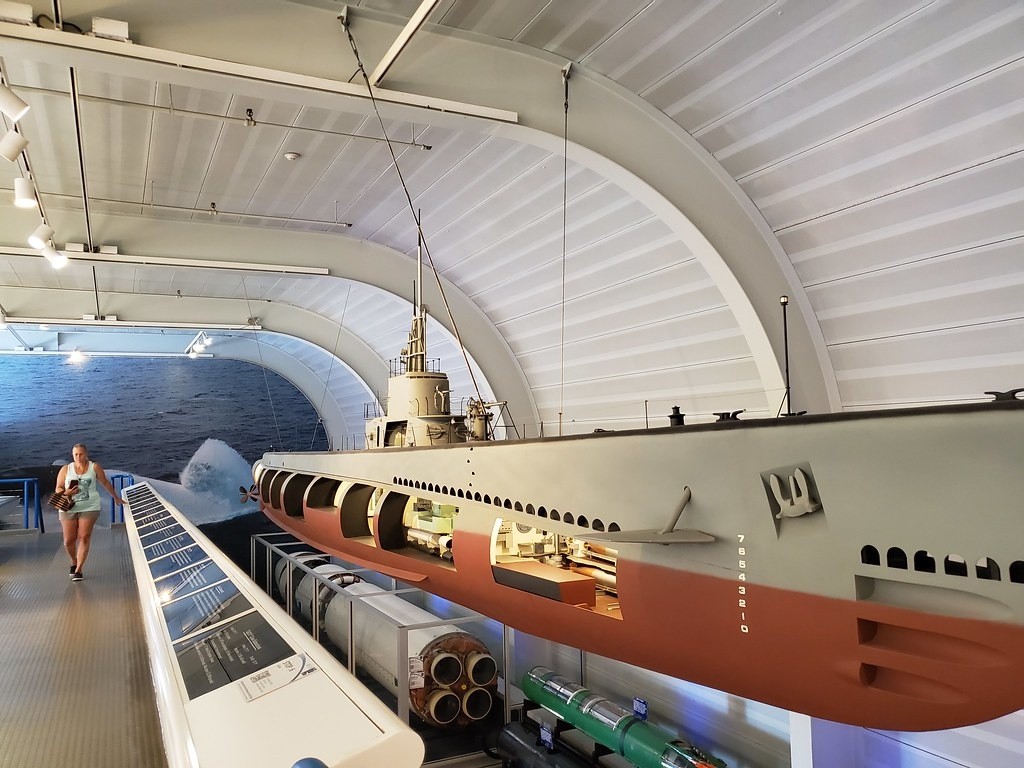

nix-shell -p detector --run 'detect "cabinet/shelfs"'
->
[493,560,598,608]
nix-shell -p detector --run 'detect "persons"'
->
[55,444,126,580]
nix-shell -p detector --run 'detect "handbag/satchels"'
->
[47,490,76,512]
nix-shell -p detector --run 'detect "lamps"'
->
[185,329,216,360]
[27,217,70,269]
[13,173,38,209]
[0,82,31,121]
[0,127,30,163]
[0,308,6,325]
[71,346,83,360]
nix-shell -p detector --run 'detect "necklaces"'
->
[77,469,85,481]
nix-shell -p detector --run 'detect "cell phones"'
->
[69,480,79,489]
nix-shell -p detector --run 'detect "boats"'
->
[238,206,1024,734]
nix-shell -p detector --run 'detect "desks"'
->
[522,552,555,563]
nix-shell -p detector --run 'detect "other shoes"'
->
[68,558,77,576]
[72,572,84,581]
